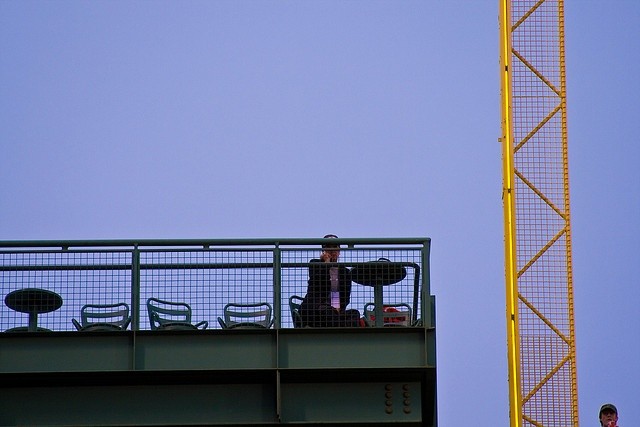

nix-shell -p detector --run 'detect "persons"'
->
[599,404,619,427]
[300,235,360,326]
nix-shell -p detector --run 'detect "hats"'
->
[599,402,618,411]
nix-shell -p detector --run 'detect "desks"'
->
[5,287,64,332]
[350,262,407,329]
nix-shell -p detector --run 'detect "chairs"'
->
[288,294,307,327]
[217,302,276,328]
[363,301,422,328]
[72,303,133,333]
[145,298,208,332]
